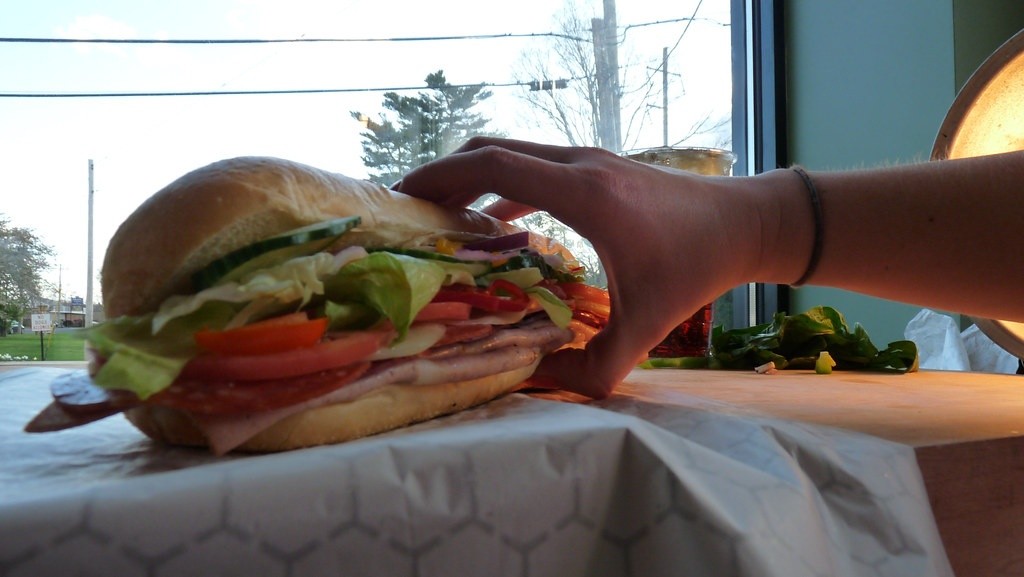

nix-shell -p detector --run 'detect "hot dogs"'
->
[24,157,611,455]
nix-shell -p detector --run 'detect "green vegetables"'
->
[639,307,921,374]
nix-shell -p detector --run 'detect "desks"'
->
[0,367,1024,577]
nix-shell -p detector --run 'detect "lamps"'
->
[929,31,1024,364]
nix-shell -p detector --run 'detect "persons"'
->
[390,134,1024,405]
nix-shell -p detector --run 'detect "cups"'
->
[617,142,738,372]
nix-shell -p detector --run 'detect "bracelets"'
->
[789,165,824,288]
